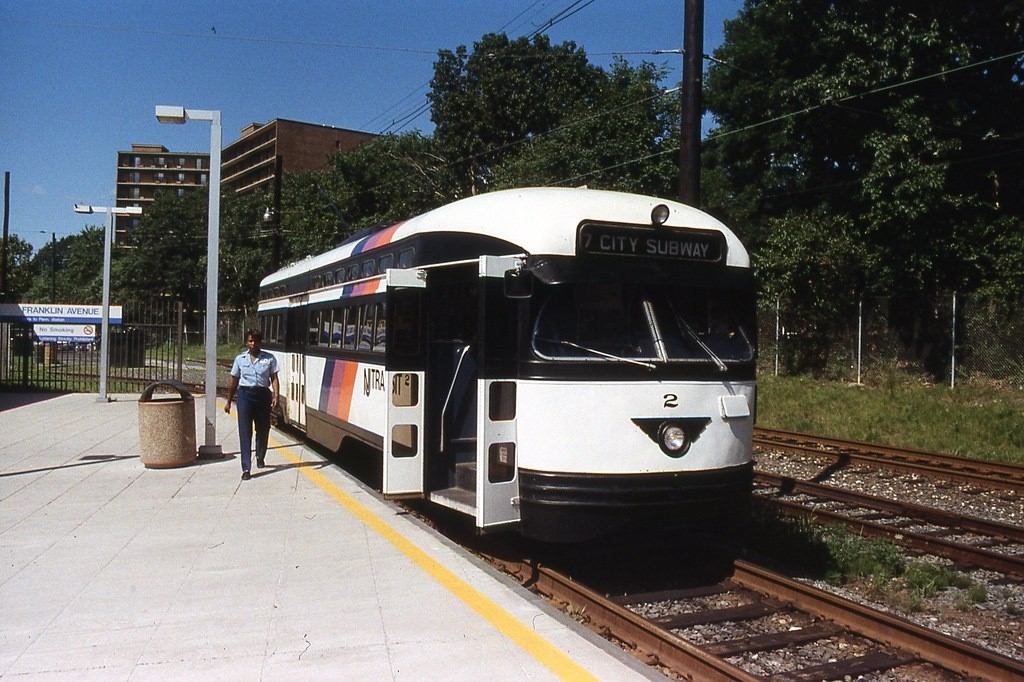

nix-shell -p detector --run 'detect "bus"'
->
[257,186,758,547]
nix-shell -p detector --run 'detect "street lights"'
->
[73,204,143,404]
[154,104,224,460]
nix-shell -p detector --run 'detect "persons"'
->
[224,329,280,480]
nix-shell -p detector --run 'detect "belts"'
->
[240,386,267,390]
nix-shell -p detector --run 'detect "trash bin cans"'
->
[136,380,198,471]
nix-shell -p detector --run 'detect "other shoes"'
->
[257,459,264,468]
[242,472,250,480]
